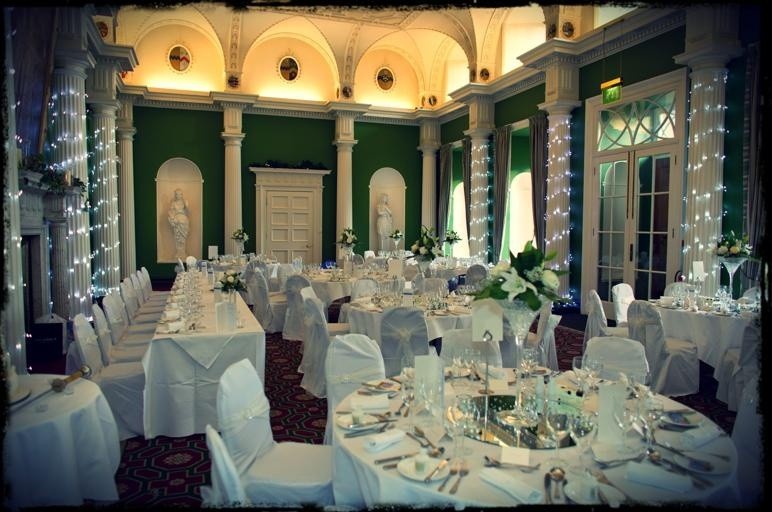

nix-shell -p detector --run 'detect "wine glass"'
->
[670,286,759,314]
[368,280,477,312]
[398,347,664,462]
[429,253,483,268]
[303,247,413,280]
[181,264,204,333]
[212,252,271,265]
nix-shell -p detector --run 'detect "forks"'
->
[482,453,542,474]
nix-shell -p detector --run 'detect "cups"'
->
[660,295,674,307]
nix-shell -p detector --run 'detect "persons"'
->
[168,189,191,258]
[377,193,393,251]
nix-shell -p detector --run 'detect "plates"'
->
[560,476,628,505]
[658,411,707,428]
[369,379,400,393]
[337,414,378,430]
[675,450,729,477]
[398,454,452,483]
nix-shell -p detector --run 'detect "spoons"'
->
[344,423,394,438]
[654,439,715,471]
[395,394,415,417]
[438,458,475,497]
[407,426,445,458]
[550,466,565,501]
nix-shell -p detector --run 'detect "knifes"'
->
[424,456,454,485]
[375,450,419,466]
[653,457,713,491]
[543,472,552,507]
